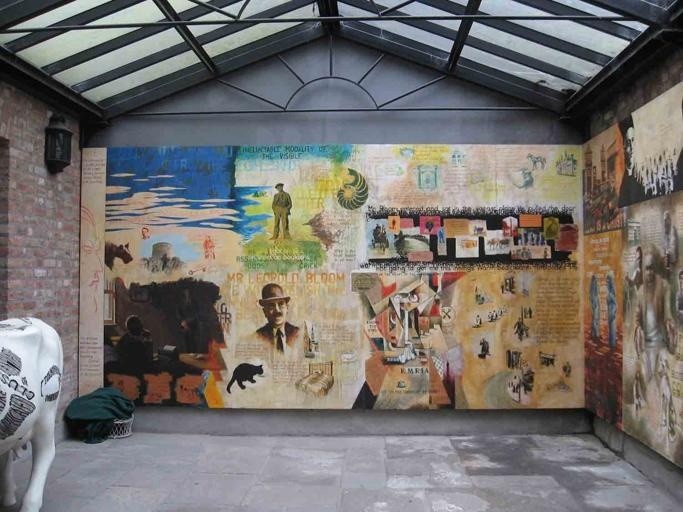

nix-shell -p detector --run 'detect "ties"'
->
[276,329,283,352]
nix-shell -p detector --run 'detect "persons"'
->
[618,118,682,427]
[604,275,617,347]
[254,282,298,356]
[141,227,151,239]
[512,317,527,341]
[203,235,215,259]
[479,337,490,355]
[271,182,292,241]
[511,231,550,260]
[588,275,601,338]
[115,314,162,377]
[177,287,200,354]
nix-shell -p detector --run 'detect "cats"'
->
[226,363,264,394]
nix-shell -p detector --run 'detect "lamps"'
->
[45,113,74,174]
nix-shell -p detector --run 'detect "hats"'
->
[258,283,289,305]
